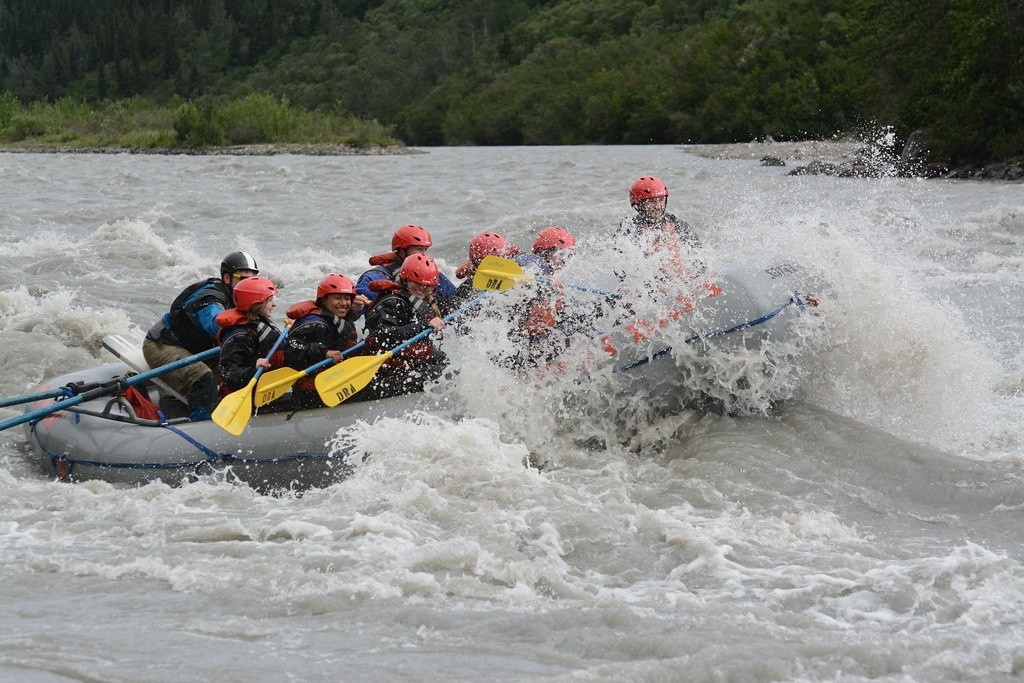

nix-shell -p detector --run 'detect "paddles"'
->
[254,339,367,407]
[211,319,297,435]
[0,342,224,431]
[314,291,489,409]
[474,254,618,298]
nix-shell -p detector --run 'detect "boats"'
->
[25,254,808,497]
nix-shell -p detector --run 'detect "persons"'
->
[142,251,260,418]
[286,273,375,407]
[216,277,301,412]
[352,224,576,396]
[614,175,704,281]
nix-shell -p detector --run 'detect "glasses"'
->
[232,274,246,282]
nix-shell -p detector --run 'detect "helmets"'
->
[532,227,576,259]
[317,274,356,304]
[392,224,432,252]
[629,175,669,207]
[400,255,438,285]
[220,251,259,278]
[470,232,506,266]
[233,277,276,312]
